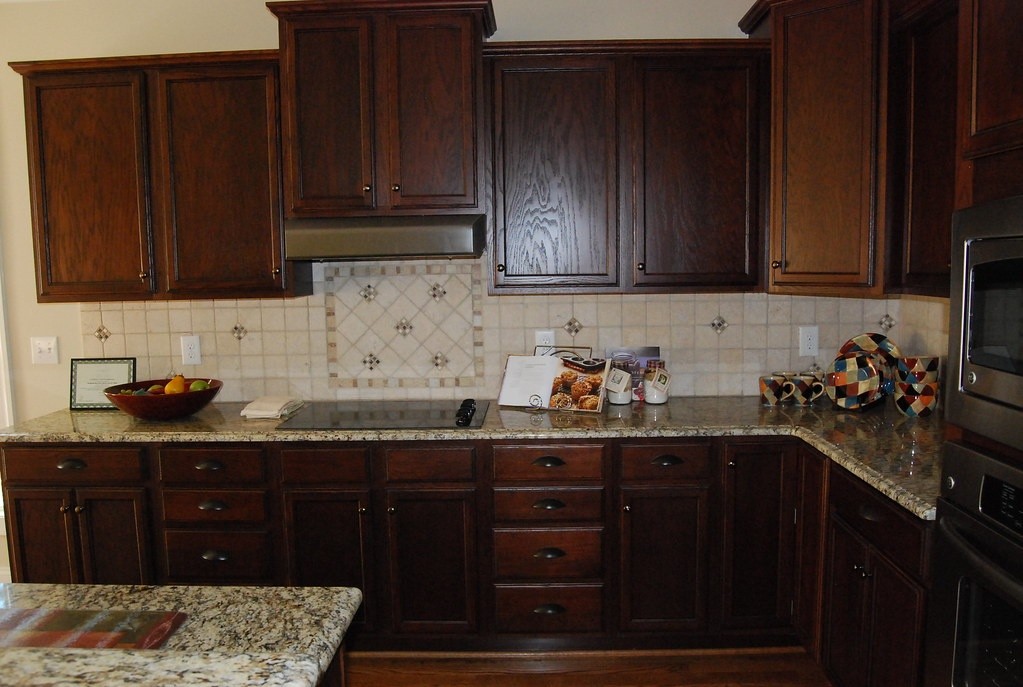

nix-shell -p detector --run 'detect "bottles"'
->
[606,359,632,405]
[643,359,669,405]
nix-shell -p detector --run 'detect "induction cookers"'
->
[275,397,491,431]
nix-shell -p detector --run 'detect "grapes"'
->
[118,389,134,395]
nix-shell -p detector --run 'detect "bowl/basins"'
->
[894,370,939,383]
[103,378,224,422]
[894,381,938,396]
[895,354,939,372]
[893,393,938,418]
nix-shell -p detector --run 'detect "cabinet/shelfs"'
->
[265,0,497,219]
[736,0,901,294]
[7,49,315,302]
[895,0,1023,300]
[0,436,1023,687]
[484,38,771,294]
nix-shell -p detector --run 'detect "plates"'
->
[824,332,905,409]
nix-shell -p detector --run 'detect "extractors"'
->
[283,214,487,263]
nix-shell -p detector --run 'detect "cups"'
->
[758,376,795,406]
[802,371,827,400]
[790,375,825,407]
[771,370,797,402]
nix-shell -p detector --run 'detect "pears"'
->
[163,372,185,393]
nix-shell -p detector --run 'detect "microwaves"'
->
[944,196,1023,451]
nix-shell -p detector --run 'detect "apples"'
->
[189,380,210,391]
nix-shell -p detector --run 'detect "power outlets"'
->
[180,335,201,365]
[30,337,59,365]
[534,330,555,357]
[798,326,819,356]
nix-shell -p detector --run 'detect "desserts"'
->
[550,371,604,411]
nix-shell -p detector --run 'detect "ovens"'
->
[921,437,1022,687]
